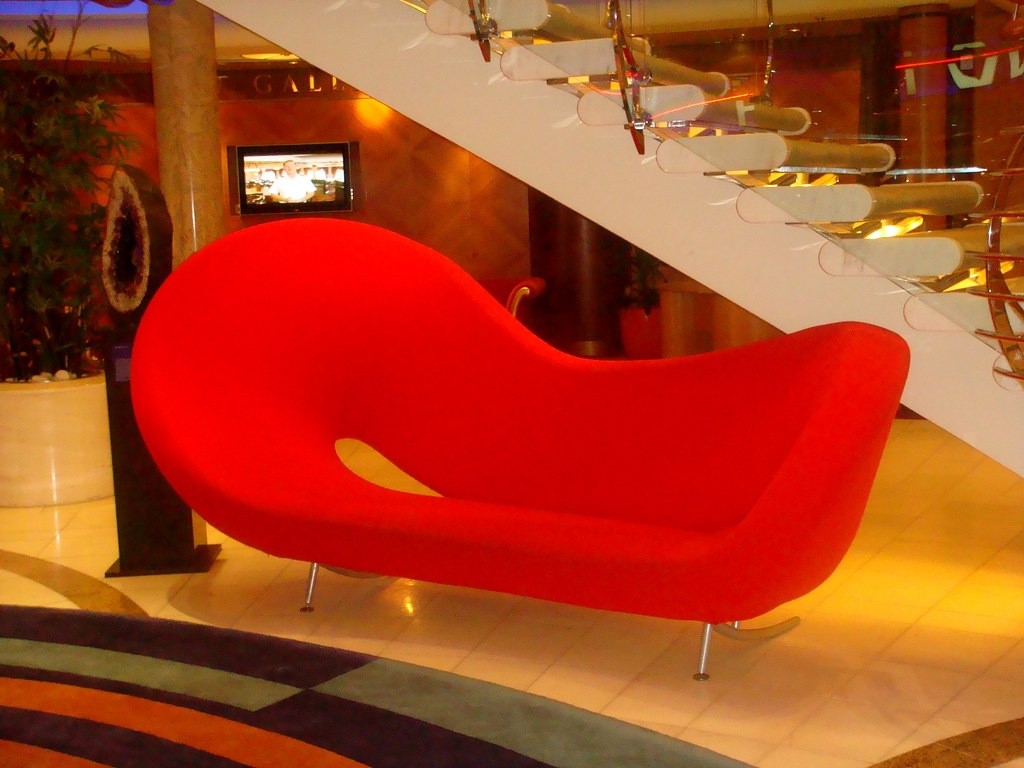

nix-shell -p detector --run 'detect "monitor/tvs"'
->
[236,141,353,215]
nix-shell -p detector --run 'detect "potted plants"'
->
[0,9,159,508]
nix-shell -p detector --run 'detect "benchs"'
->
[128,215,912,682]
